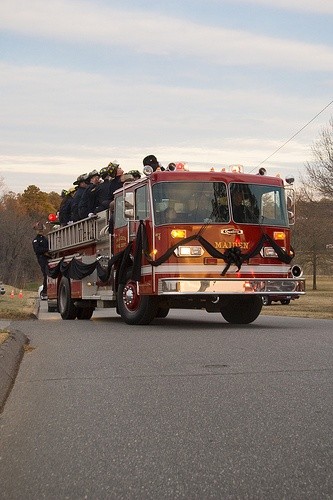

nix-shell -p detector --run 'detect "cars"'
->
[38,285,50,301]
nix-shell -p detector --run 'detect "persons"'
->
[33,155,258,225]
[32,222,53,297]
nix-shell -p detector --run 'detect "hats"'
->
[73,162,119,184]
[143,155,159,171]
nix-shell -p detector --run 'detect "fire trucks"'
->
[32,155,307,325]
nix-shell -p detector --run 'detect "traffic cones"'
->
[10,290,15,299]
[18,289,23,299]
